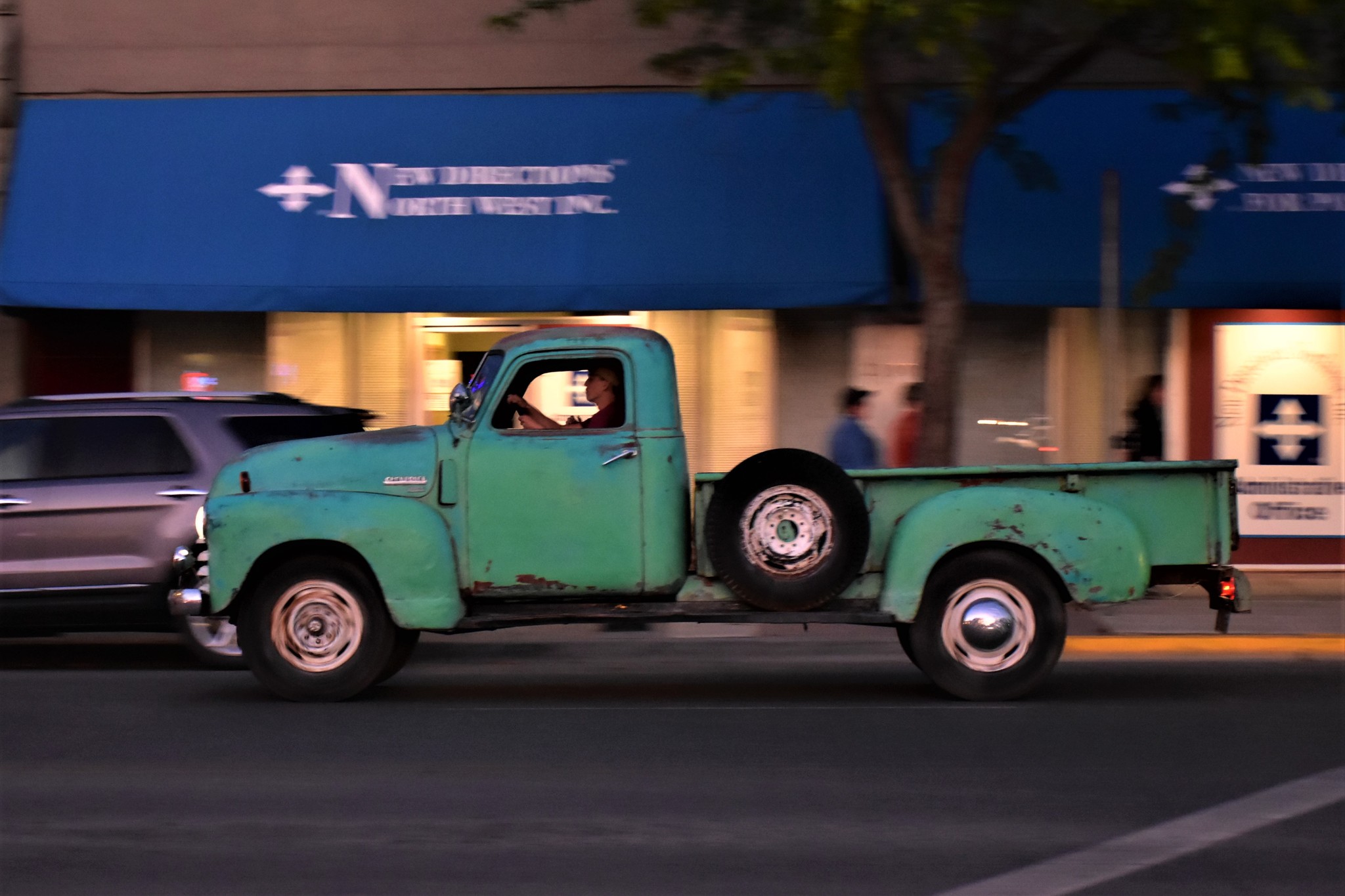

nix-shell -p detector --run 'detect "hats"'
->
[585,357,624,389]
[841,388,871,406]
[907,384,932,400]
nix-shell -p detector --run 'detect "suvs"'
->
[0,392,370,663]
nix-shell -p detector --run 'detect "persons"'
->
[833,382,959,468]
[1109,374,1166,464]
[506,355,626,432]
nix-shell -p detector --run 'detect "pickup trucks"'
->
[201,327,1250,704]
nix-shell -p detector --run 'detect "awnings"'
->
[0,83,1345,312]
